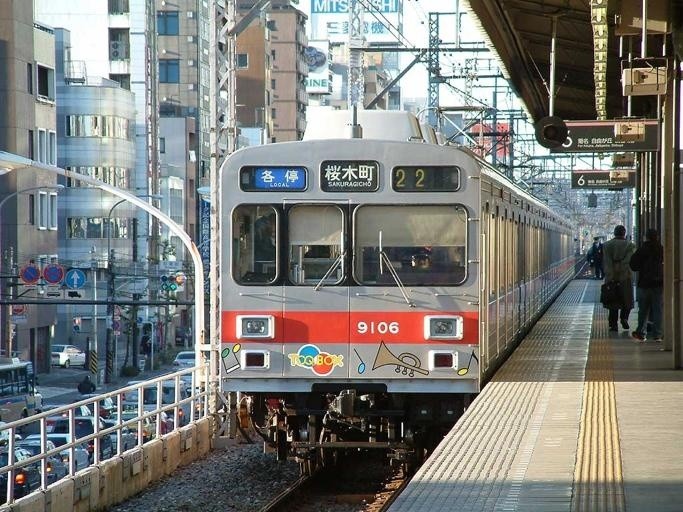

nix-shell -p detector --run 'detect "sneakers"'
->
[609,326,619,332]
[632,331,648,342]
[620,320,630,330]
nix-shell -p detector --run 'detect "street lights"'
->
[0,183,65,206]
[106,192,164,261]
[1,146,206,412]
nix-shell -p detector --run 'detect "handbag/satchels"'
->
[600,279,635,311]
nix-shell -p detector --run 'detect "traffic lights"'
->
[166,275,177,292]
[175,274,186,285]
[159,275,168,291]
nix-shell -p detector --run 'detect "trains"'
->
[214,103,594,488]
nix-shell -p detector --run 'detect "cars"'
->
[0,343,212,506]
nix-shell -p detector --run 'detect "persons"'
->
[76,375,97,394]
[254,212,274,275]
[408,248,434,274]
[600,224,636,332]
[591,235,605,281]
[585,246,594,277]
[628,228,664,344]
